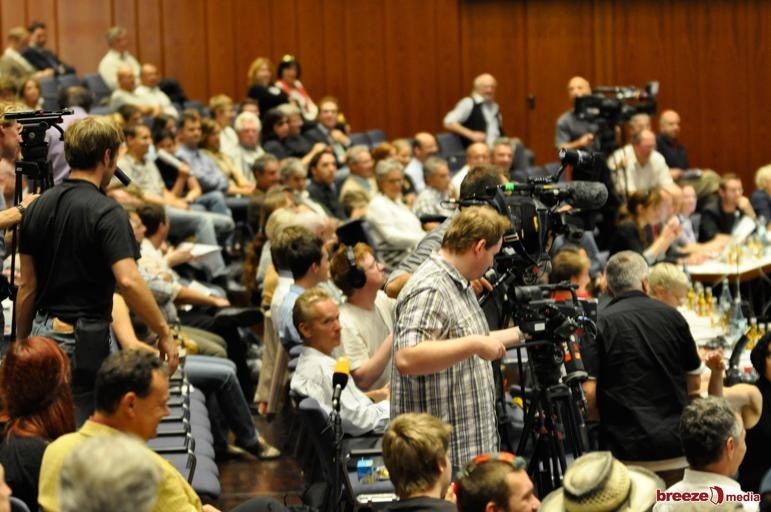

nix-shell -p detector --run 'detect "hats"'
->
[538,451,666,512]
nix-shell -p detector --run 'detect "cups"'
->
[730,235,765,265]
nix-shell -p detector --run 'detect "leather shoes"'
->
[215,307,263,328]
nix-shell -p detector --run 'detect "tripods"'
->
[492,298,585,496]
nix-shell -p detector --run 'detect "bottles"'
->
[688,274,771,375]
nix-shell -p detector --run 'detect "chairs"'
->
[142,347,529,512]
[34,65,569,187]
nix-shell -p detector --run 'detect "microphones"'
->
[332,357,350,406]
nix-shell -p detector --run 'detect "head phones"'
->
[344,245,367,289]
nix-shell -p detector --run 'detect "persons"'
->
[2,19,771,512]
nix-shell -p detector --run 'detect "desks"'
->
[676,241,771,292]
